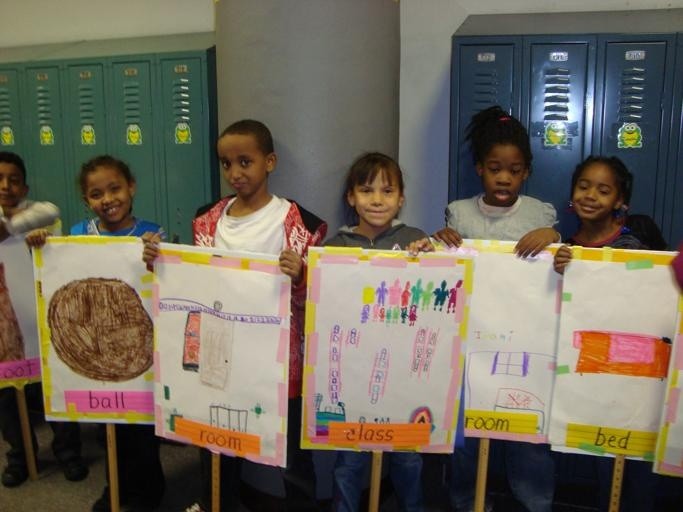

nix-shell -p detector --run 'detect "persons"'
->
[25,156,167,511]
[554,156,651,510]
[432,104,563,511]
[143,120,327,511]
[0,149,84,486]
[321,151,434,511]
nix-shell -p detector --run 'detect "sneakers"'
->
[61,460,88,480]
[4,465,28,486]
[92,488,112,512]
[184,502,204,512]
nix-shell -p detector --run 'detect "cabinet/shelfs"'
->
[451,31,683,252]
[0,31,214,245]
